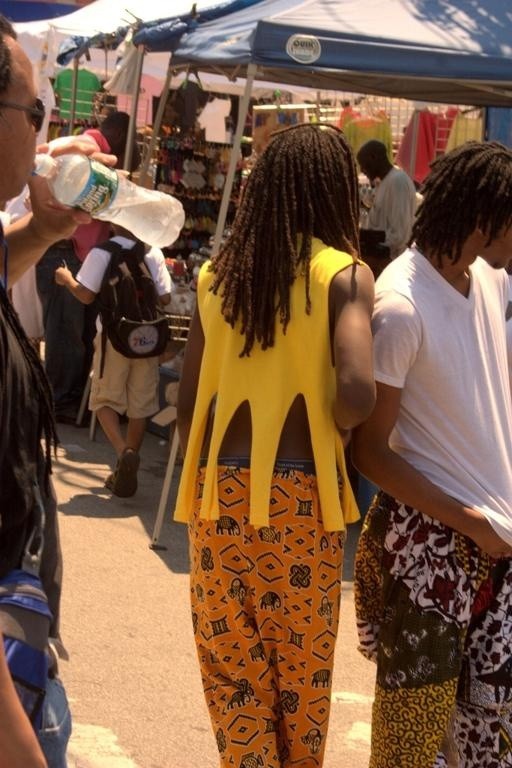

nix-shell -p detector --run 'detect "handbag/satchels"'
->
[1,568,54,733]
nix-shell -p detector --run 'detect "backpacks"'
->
[94,239,168,359]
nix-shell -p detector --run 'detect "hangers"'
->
[341,95,391,137]
[49,122,84,135]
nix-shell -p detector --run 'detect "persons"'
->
[36,111,171,428]
[0,15,71,768]
[350,141,512,768]
[56,222,172,496]
[357,140,415,259]
[176,123,377,768]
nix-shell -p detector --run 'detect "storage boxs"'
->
[151,405,184,459]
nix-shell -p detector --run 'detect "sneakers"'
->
[102,447,139,498]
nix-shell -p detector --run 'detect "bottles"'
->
[32,152,185,250]
[356,182,378,216]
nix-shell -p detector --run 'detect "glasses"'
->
[0,98,46,133]
